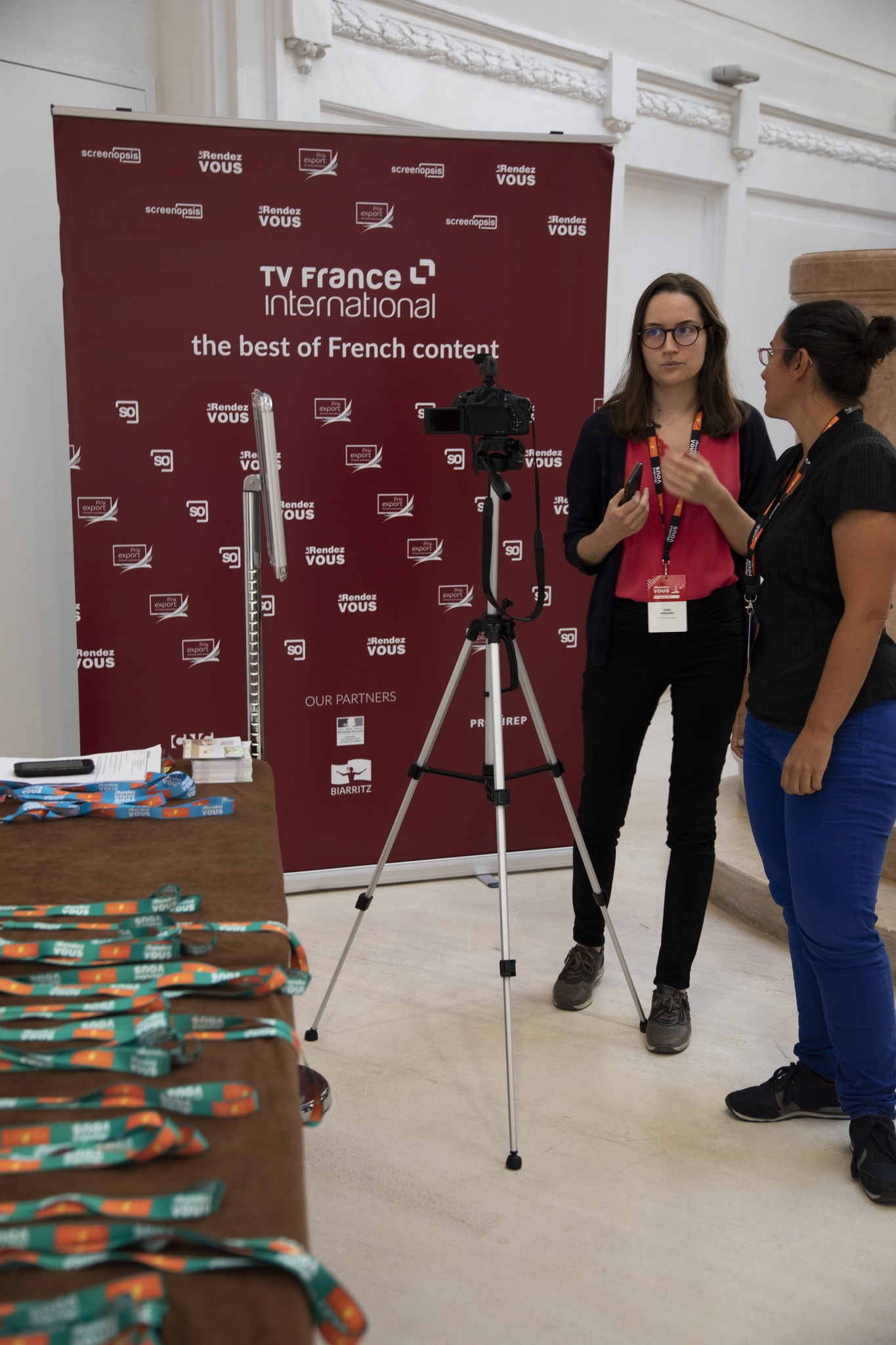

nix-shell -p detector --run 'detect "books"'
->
[181,735,255,783]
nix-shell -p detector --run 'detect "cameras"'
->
[424,354,534,500]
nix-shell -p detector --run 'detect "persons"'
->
[555,274,781,1055]
[727,296,896,1211]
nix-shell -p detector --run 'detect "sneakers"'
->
[645,984,691,1052]
[553,944,605,1010]
[849,1114,896,1202]
[725,1061,850,1122]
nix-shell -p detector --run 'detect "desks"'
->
[2,753,317,1344]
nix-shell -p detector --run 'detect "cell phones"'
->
[14,759,94,777]
[622,461,643,505]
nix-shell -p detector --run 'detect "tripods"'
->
[305,471,648,1172]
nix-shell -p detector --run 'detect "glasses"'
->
[638,324,705,349]
[757,346,813,366]
